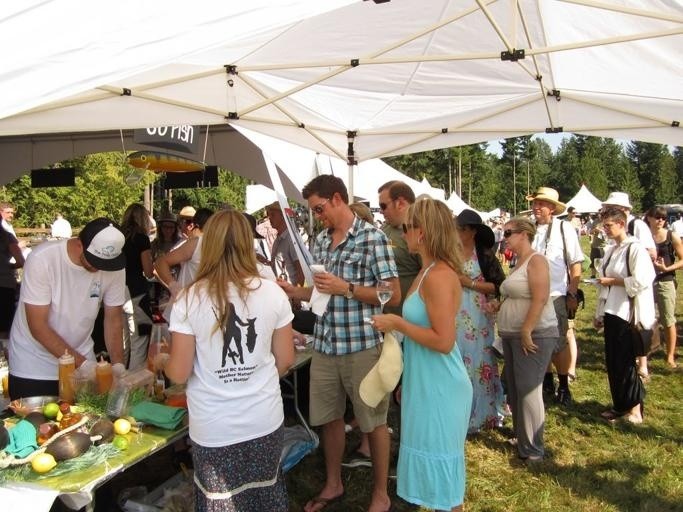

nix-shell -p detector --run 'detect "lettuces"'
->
[73,387,148,420]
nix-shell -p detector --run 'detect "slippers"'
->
[599,409,622,420]
[607,416,635,427]
[668,362,677,369]
[303,492,348,512]
[637,369,648,379]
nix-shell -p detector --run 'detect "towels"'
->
[4,419,39,459]
[129,402,188,431]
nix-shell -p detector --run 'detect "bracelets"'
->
[471,281,476,293]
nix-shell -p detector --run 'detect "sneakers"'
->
[525,455,544,467]
[557,389,571,406]
[341,450,372,467]
[544,387,556,403]
[501,438,518,448]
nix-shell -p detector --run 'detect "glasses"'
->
[572,212,577,214]
[655,216,668,220]
[379,198,395,210]
[402,222,420,233]
[531,191,545,198]
[458,225,468,232]
[311,198,329,214]
[603,224,615,229]
[503,229,521,237]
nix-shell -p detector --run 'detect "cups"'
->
[594,258,604,277]
[376,279,393,316]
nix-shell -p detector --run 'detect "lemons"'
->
[31,454,56,474]
[114,418,131,433]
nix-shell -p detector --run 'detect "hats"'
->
[601,193,632,211]
[155,212,178,224]
[179,206,196,218]
[457,209,496,251]
[525,187,566,216]
[242,213,265,240]
[359,331,403,408]
[80,217,127,272]
[265,201,281,211]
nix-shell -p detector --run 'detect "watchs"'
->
[565,291,580,298]
[345,282,355,301]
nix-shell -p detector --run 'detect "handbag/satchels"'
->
[560,221,585,320]
[626,242,661,356]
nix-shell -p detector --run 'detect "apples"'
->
[44,402,59,418]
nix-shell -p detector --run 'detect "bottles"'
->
[95,355,113,393]
[59,348,76,405]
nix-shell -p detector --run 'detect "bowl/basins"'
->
[8,395,60,409]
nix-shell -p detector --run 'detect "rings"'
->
[320,284,323,288]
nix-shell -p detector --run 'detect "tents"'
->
[1,3,683,165]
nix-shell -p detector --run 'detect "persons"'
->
[180,205,195,234]
[589,208,608,279]
[349,203,375,226]
[8,216,126,398]
[275,174,402,512]
[526,187,584,404]
[0,201,29,264]
[172,207,215,251]
[482,216,560,468]
[455,208,507,439]
[51,211,72,239]
[602,191,658,384]
[145,210,157,234]
[642,206,682,368]
[370,198,473,512]
[671,212,683,257]
[583,209,657,426]
[337,181,415,479]
[153,206,296,512]
[155,212,265,345]
[150,201,183,312]
[119,202,155,370]
[484,206,508,264]
[1,213,26,332]
[563,206,582,239]
[256,200,304,311]
